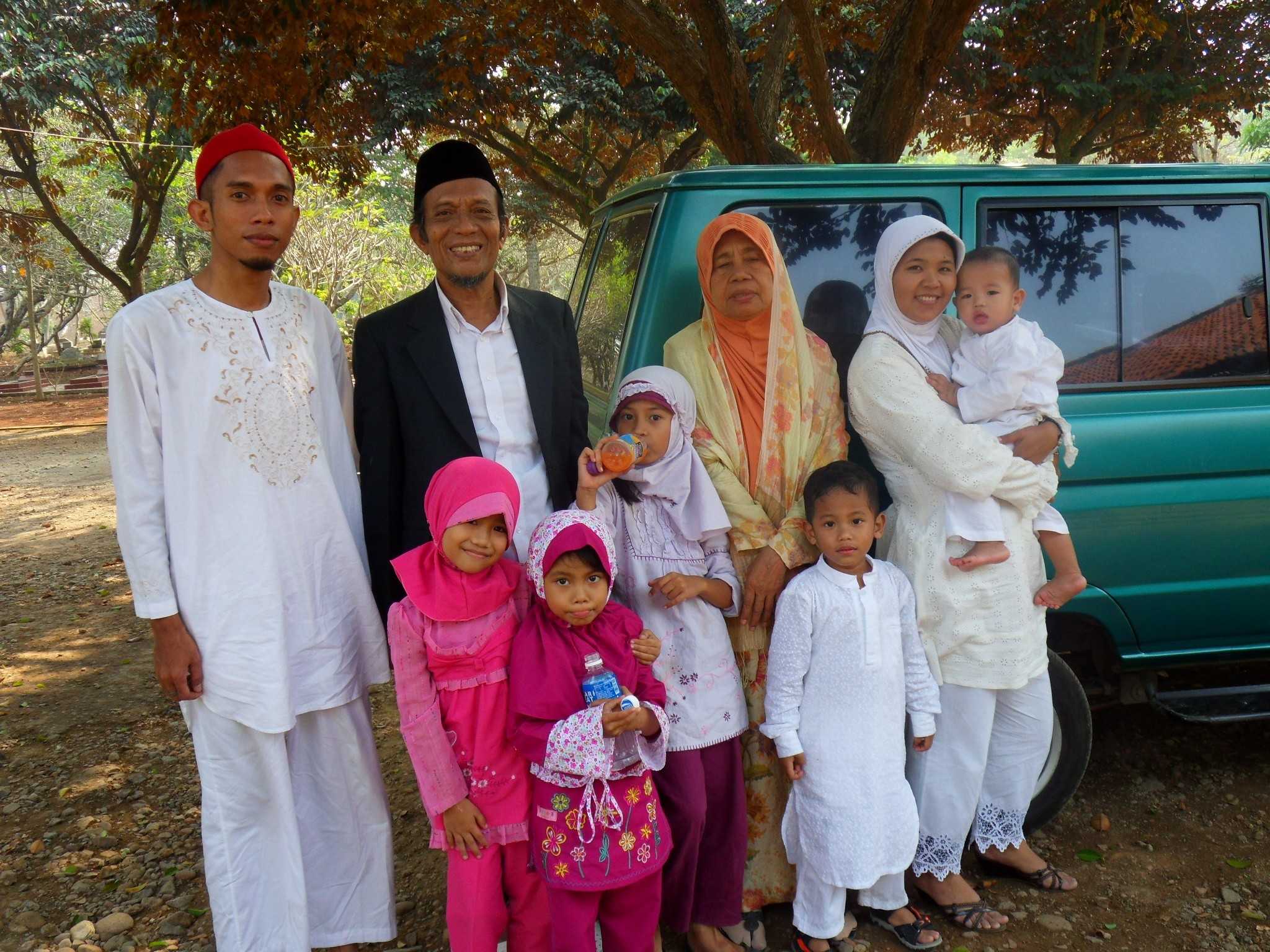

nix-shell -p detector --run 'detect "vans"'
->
[564,161,1270,839]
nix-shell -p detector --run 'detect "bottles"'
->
[600,434,648,472]
[581,651,641,770]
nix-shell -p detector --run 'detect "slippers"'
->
[912,882,1007,932]
[975,852,1072,892]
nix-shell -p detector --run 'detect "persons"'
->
[388,457,661,952]
[106,124,398,952]
[354,140,590,625]
[573,365,753,952]
[663,210,853,946]
[508,510,671,952]
[846,214,1088,929]
[758,460,943,951]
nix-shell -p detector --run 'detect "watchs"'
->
[1039,416,1064,447]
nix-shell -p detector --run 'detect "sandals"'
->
[791,928,838,952]
[872,901,943,949]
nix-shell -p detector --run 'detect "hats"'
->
[414,140,508,211]
[195,123,300,195]
